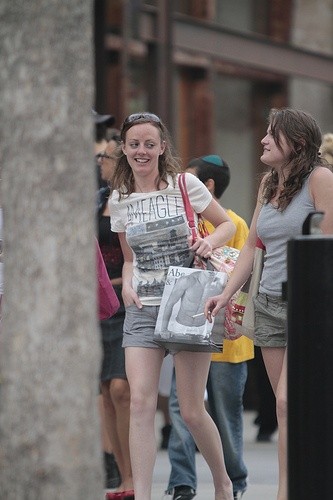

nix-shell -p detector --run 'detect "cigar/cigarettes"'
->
[192,310,211,318]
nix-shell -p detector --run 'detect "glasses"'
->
[128,111,161,123]
[95,153,118,164]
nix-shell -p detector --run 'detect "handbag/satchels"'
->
[152,265,224,353]
[178,172,255,341]
[240,236,267,340]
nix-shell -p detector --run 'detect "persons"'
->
[203,107,333,500]
[254,133,333,441]
[164,153,255,500]
[95,111,208,500]
[107,113,237,500]
[160,270,226,344]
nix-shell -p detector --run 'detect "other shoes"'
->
[233,488,246,500]
[172,485,195,500]
[106,490,134,500]
